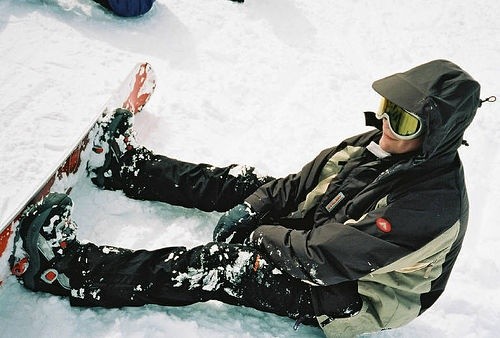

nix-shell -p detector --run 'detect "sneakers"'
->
[12,192,80,295]
[86,108,140,191]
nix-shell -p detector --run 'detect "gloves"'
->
[213,203,256,244]
[228,232,250,244]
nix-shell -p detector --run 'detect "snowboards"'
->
[0,58,157,283]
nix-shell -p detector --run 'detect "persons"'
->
[11,59,481,338]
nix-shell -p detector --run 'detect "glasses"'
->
[375,95,424,141]
